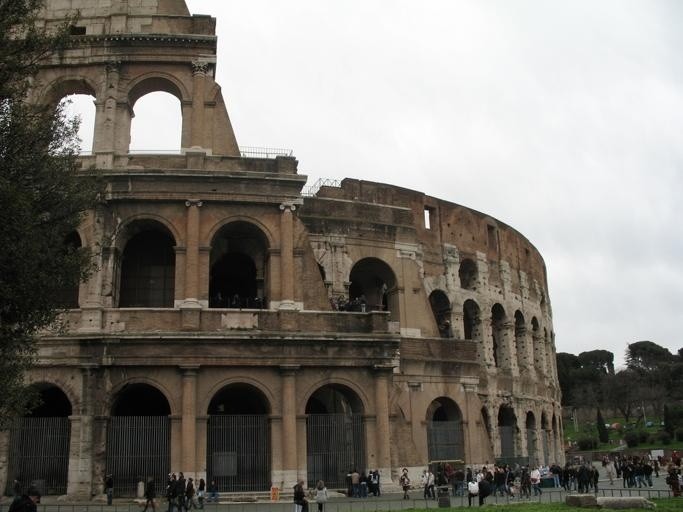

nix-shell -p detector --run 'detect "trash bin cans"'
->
[437,487,450,507]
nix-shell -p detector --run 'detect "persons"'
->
[345,469,380,497]
[142,471,219,511]
[420,448,683,507]
[398,468,412,498]
[101,473,114,506]
[291,478,312,511]
[211,290,224,307]
[231,293,241,307]
[311,480,329,511]
[10,475,23,497]
[336,291,364,311]
[7,485,41,511]
